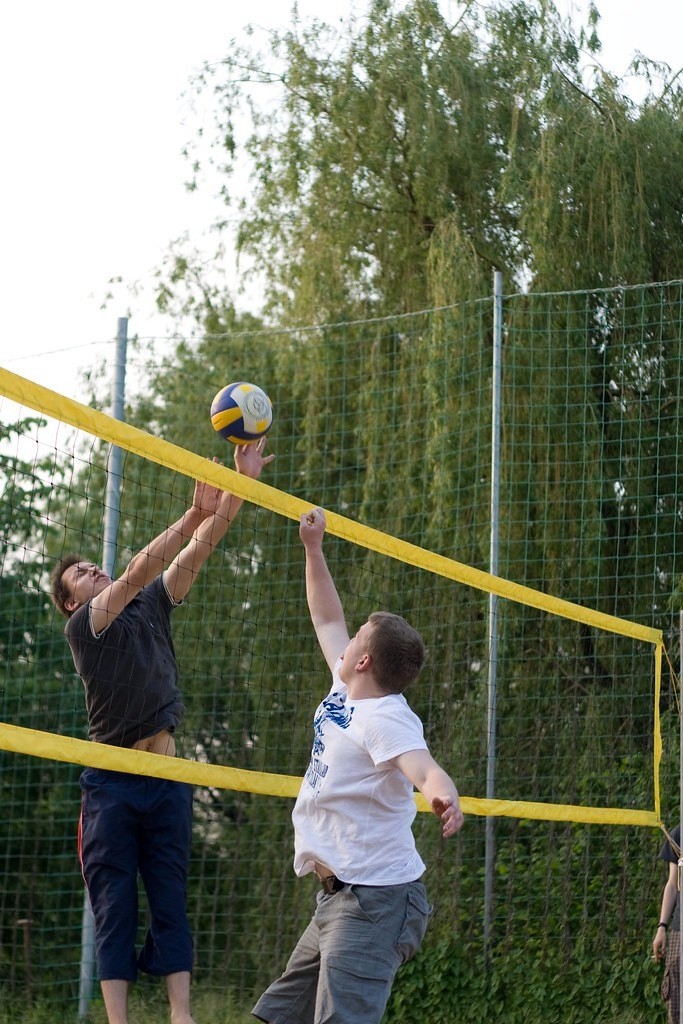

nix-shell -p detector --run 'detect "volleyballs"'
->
[210,381,274,446]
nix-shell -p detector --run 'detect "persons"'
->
[652,826,683,1024]
[51,434,277,1024]
[249,505,463,1024]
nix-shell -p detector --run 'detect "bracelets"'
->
[657,923,668,932]
